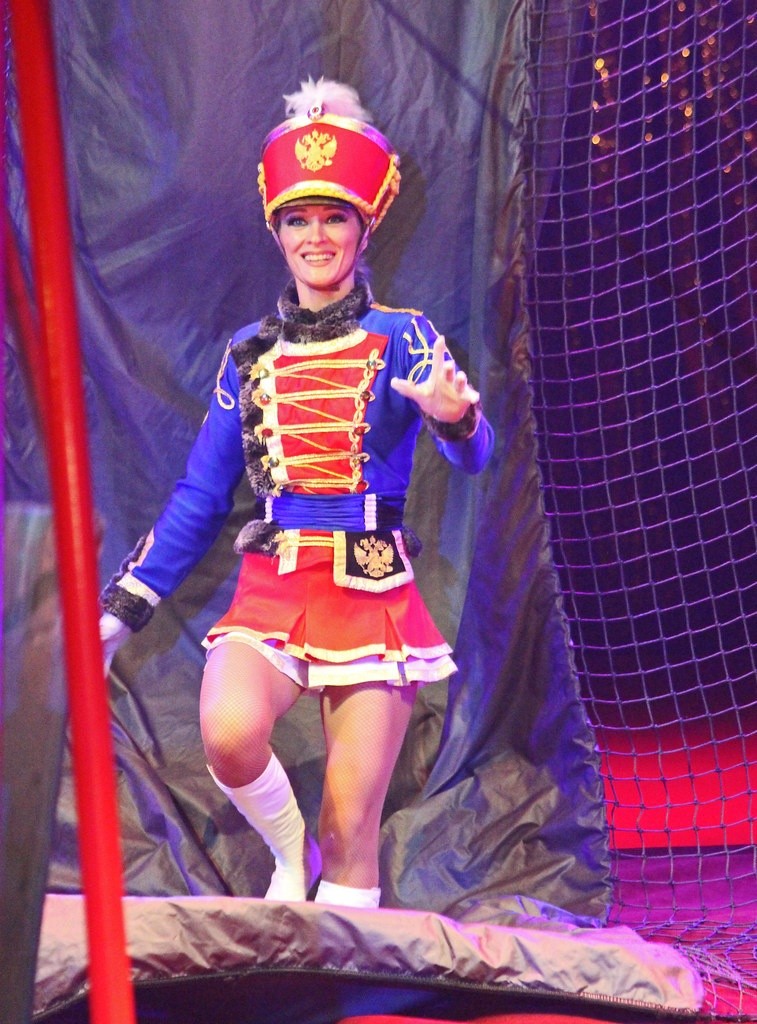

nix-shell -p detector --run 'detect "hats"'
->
[257,76,403,235]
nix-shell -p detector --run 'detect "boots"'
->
[206,749,323,902]
[314,880,383,907]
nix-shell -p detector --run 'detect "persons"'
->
[96,76,494,908]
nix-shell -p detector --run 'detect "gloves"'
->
[390,334,480,422]
[101,612,131,678]
